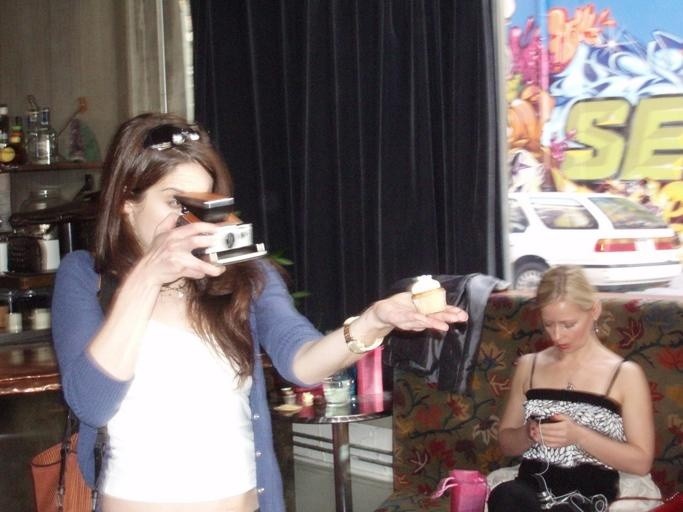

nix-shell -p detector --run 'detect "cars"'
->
[505,190,682,294]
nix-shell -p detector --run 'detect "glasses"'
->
[137,123,221,153]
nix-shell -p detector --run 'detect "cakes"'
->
[410,275,446,314]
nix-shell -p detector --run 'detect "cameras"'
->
[174,191,269,266]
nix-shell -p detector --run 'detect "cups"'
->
[320,377,352,406]
[5,308,51,332]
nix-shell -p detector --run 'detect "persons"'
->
[481,261,666,511]
[48,112,471,511]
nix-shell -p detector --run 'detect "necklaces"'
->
[158,284,184,299]
[554,347,594,392]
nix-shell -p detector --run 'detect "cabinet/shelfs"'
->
[1,158,112,343]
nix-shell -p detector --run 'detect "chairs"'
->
[369,288,683,511]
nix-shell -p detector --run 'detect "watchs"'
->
[340,312,387,354]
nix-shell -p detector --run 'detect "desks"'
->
[264,386,393,512]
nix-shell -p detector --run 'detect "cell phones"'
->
[530,413,550,423]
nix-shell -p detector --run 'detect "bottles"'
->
[19,189,67,213]
[37,214,79,271]
[356,344,384,413]
[0,95,59,166]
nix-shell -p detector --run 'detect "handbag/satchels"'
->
[431,470,492,511]
[610,490,683,512]
[29,409,102,512]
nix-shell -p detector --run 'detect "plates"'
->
[273,404,302,411]
